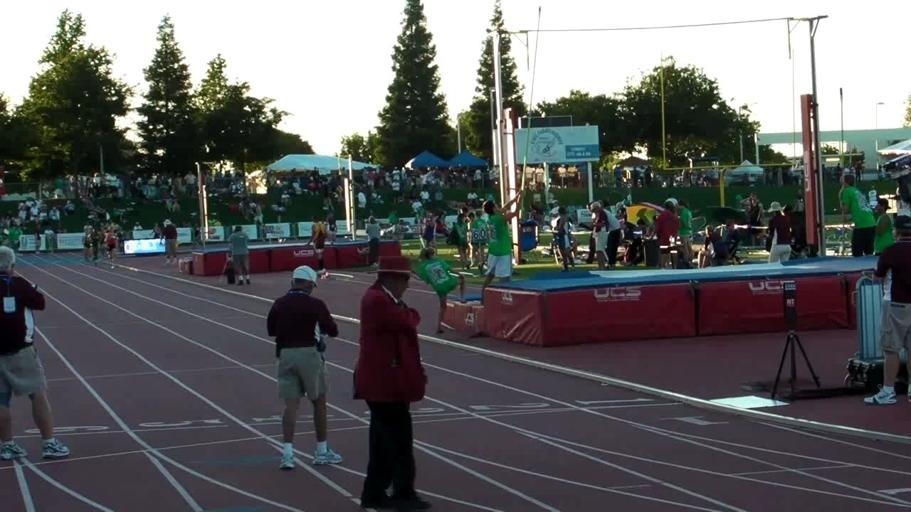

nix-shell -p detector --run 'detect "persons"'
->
[353,257,433,512]
[864,216,911,404]
[0,246,70,460]
[267,265,343,469]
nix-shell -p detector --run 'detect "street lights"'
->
[740,105,748,166]
[876,102,885,180]
[661,55,674,169]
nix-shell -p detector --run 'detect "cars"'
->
[0,160,267,203]
[616,161,723,187]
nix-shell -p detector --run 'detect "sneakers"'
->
[360,491,430,512]
[42,439,70,457]
[280,454,294,468]
[864,385,897,405]
[313,449,342,464]
[0,444,27,460]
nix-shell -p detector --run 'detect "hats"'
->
[368,256,414,274]
[768,202,781,213]
[293,266,318,288]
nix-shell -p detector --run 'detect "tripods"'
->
[770,307,821,399]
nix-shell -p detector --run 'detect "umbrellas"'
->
[877,139,911,160]
[732,159,762,175]
[449,150,489,168]
[405,150,450,168]
